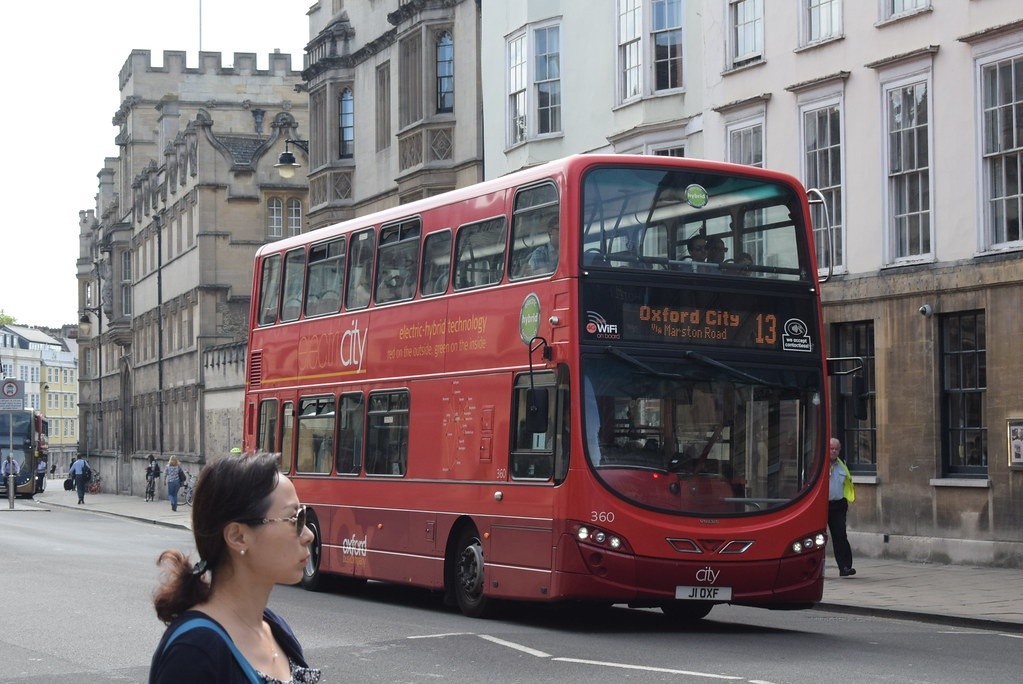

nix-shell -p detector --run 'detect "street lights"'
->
[79,306,102,402]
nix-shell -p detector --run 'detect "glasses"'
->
[711,247,728,253]
[220,505,306,537]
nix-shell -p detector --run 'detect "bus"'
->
[0,409,49,499]
[239,154,866,623]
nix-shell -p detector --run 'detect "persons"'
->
[148,454,159,501]
[968,436,987,465]
[37,459,46,493]
[68,453,90,504]
[70,458,76,491]
[682,235,721,273]
[528,218,560,274]
[146,448,322,684]
[164,455,184,510]
[563,403,605,453]
[736,253,753,275]
[706,235,745,274]
[827,438,856,576]
[1,455,20,498]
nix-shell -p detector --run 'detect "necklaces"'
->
[215,596,278,658]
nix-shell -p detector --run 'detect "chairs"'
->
[262,246,751,325]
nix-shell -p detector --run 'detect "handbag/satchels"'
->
[82,460,91,481]
[64,478,73,490]
[177,465,186,481]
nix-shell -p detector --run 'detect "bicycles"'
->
[176,471,197,507]
[88,469,104,495]
[2,474,20,503]
[145,470,163,502]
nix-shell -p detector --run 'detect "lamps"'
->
[78,307,100,335]
[273,139,308,178]
[40,381,49,393]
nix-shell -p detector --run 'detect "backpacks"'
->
[150,460,160,477]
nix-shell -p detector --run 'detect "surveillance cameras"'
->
[919,304,932,318]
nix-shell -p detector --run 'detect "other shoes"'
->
[840,565,856,576]
[78,497,84,504]
[173,505,177,511]
